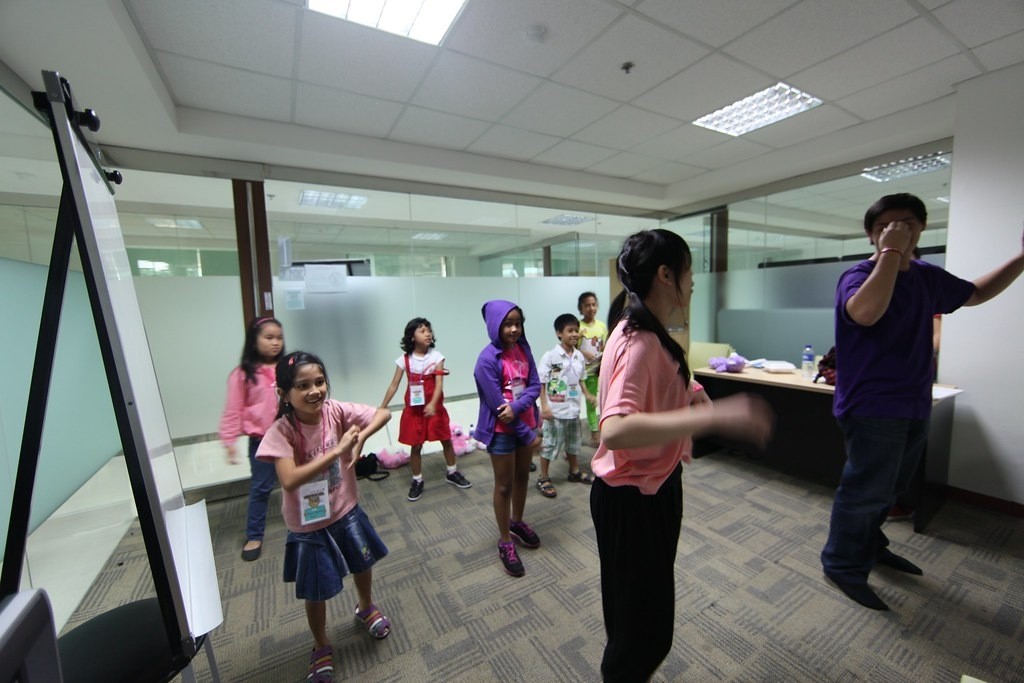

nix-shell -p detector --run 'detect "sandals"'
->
[306,644,334,683]
[354,602,392,639]
[537,474,557,498]
[567,468,596,484]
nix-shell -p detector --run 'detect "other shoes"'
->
[823,565,890,612]
[242,539,263,561]
[885,503,917,521]
[528,463,537,471]
[874,549,924,576]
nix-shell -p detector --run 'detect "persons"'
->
[255,351,391,683]
[563,291,608,458]
[220,316,285,560]
[536,313,596,498]
[822,193,1024,611]
[590,229,772,683]
[382,317,472,500]
[473,300,541,576]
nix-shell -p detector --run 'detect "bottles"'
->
[801,344,814,381]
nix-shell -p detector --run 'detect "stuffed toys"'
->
[377,447,410,468]
[470,430,487,449]
[448,424,476,455]
[709,351,749,372]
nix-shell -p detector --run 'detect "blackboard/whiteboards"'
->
[39,65,201,665]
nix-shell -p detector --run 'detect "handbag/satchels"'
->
[815,346,836,385]
[358,453,390,481]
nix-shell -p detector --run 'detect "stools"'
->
[56,596,223,683]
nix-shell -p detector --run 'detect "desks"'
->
[694,364,958,533]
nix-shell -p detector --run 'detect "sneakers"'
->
[445,471,472,488]
[498,536,525,576]
[510,519,540,547]
[408,479,424,500]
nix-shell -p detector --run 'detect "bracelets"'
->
[879,247,904,255]
